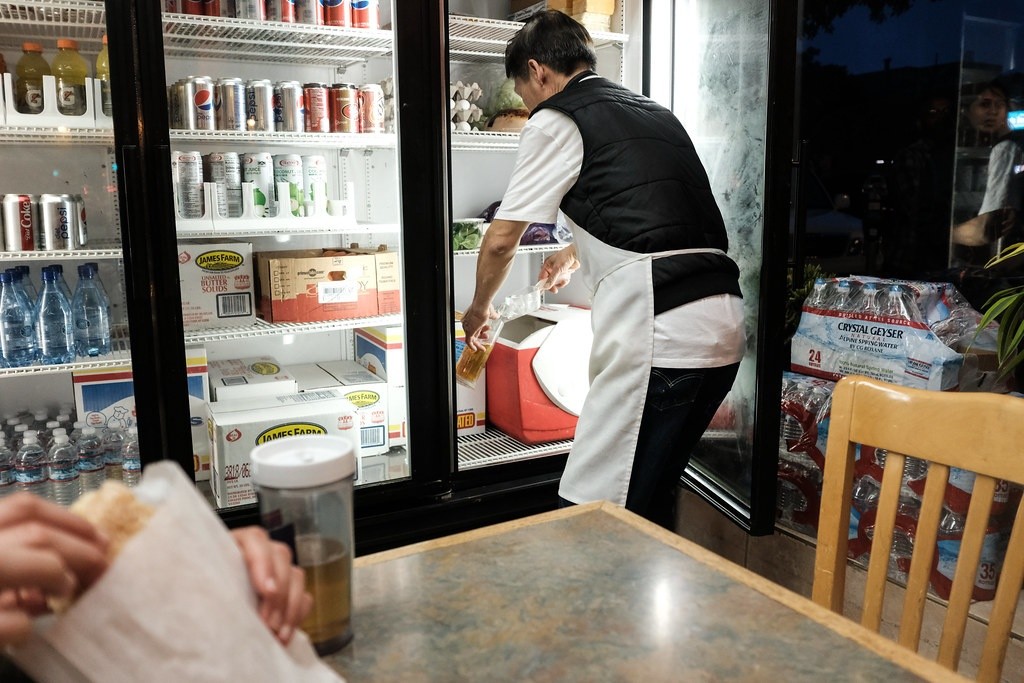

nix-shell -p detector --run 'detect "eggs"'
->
[450,98,471,132]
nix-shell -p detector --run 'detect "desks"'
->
[319,498,985,683]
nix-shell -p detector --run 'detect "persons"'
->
[459,9,749,539]
[957,81,1008,149]
[0,479,315,683]
[950,102,1024,280]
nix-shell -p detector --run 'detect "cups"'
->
[456,318,504,390]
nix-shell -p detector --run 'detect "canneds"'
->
[159,0,381,31]
[171,150,329,220]
[0,192,88,252]
[166,74,386,135]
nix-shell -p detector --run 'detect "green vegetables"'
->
[452,221,481,250]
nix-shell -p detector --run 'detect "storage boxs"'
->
[177,239,401,332]
[74,310,486,510]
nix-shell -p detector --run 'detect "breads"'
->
[544,0,616,16]
[47,479,154,613]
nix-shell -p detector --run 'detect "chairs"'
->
[811,376,1024,683]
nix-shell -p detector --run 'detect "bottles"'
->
[0,266,39,367]
[0,406,141,508]
[16,43,51,114]
[791,278,958,391]
[52,40,91,116]
[251,435,355,657]
[71,263,112,357]
[778,371,1024,601]
[0,54,8,102]
[34,264,73,365]
[351,242,387,250]
[97,35,112,117]
